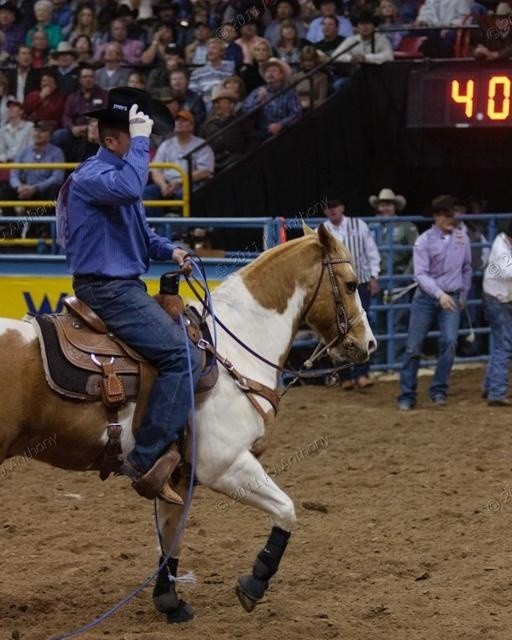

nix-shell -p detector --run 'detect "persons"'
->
[478,218,512,407]
[55,86,203,506]
[318,190,380,395]
[448,198,490,354]
[375,2,512,61]
[369,186,419,364]
[396,193,472,408]
[0,1,394,239]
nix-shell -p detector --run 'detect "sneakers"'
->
[358,375,373,387]
[431,395,447,406]
[341,380,354,391]
[399,402,410,410]
[482,389,512,407]
[119,457,184,504]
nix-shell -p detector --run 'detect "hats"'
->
[173,112,195,123]
[34,120,51,132]
[50,43,79,60]
[84,86,175,136]
[351,11,381,28]
[490,3,511,18]
[259,59,292,80]
[370,188,406,213]
[6,100,18,110]
[210,86,240,103]
[425,195,466,216]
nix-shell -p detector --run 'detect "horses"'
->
[0,218,378,624]
